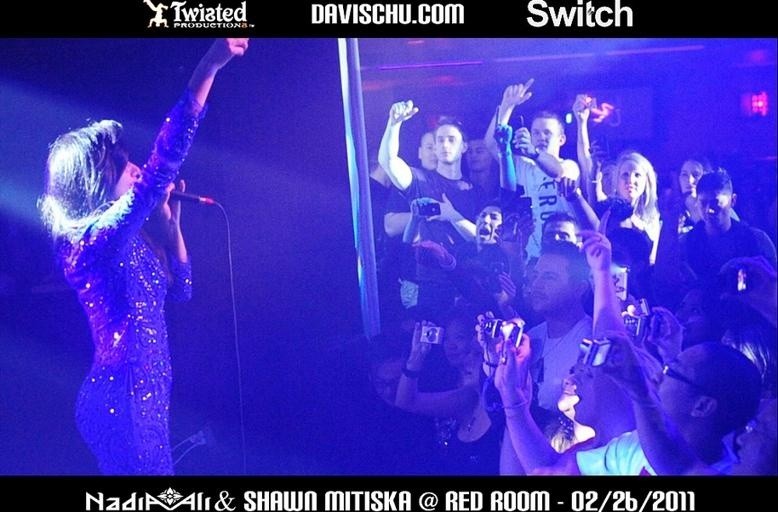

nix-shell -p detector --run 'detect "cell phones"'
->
[615,264,629,301]
[513,115,524,129]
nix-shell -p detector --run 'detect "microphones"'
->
[169,190,214,205]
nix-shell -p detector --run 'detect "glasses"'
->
[662,361,698,389]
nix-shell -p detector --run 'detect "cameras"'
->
[501,318,526,348]
[419,325,444,344]
[585,97,597,111]
[625,316,644,338]
[417,202,441,216]
[579,337,611,367]
[639,298,651,316]
[483,317,501,339]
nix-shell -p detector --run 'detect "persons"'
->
[37,37,249,474]
[363,73,778,476]
